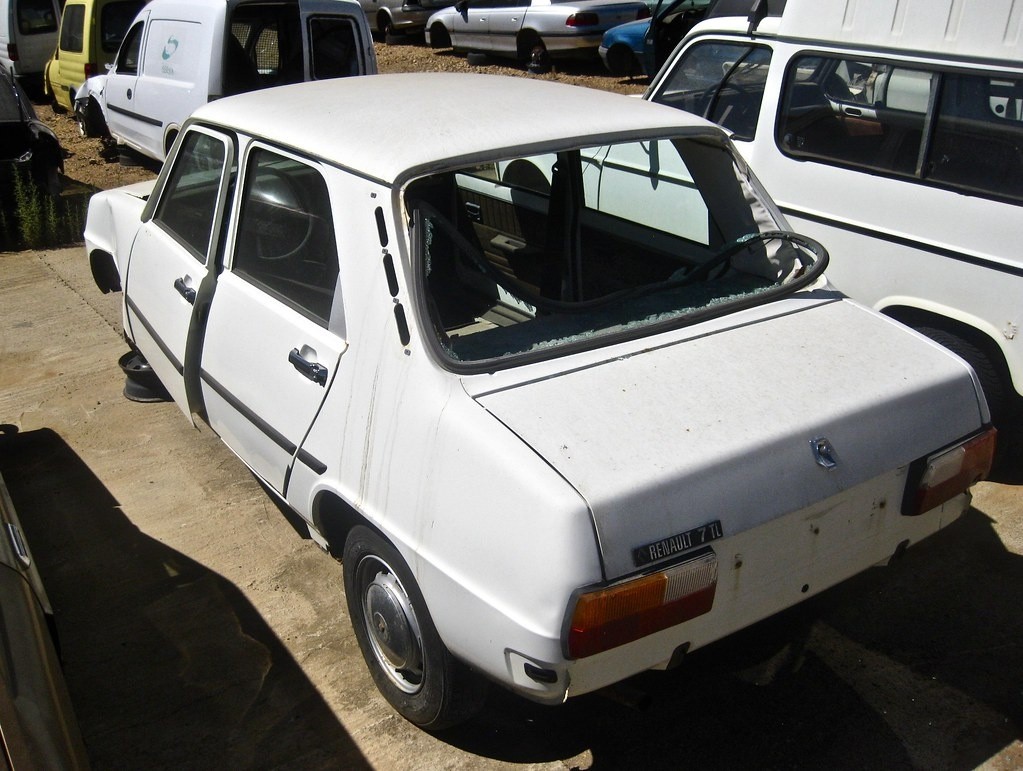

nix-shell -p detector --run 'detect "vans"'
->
[71,0,378,171]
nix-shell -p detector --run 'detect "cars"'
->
[1,0,70,79]
[422,0,652,73]
[41,0,144,120]
[83,73,997,740]
[595,0,717,78]
[356,0,463,45]
[489,18,1023,484]
